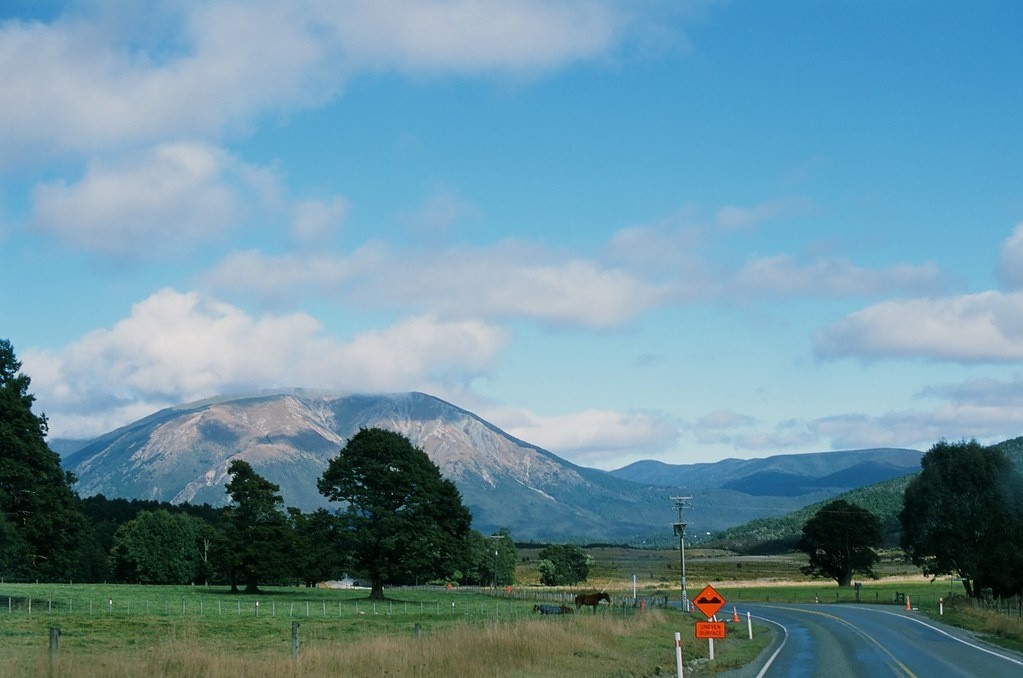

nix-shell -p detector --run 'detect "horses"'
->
[531,603,574,615]
[575,592,612,615]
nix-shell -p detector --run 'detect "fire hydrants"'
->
[640,600,645,614]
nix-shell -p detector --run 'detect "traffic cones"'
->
[905,596,911,610]
[732,605,740,622]
